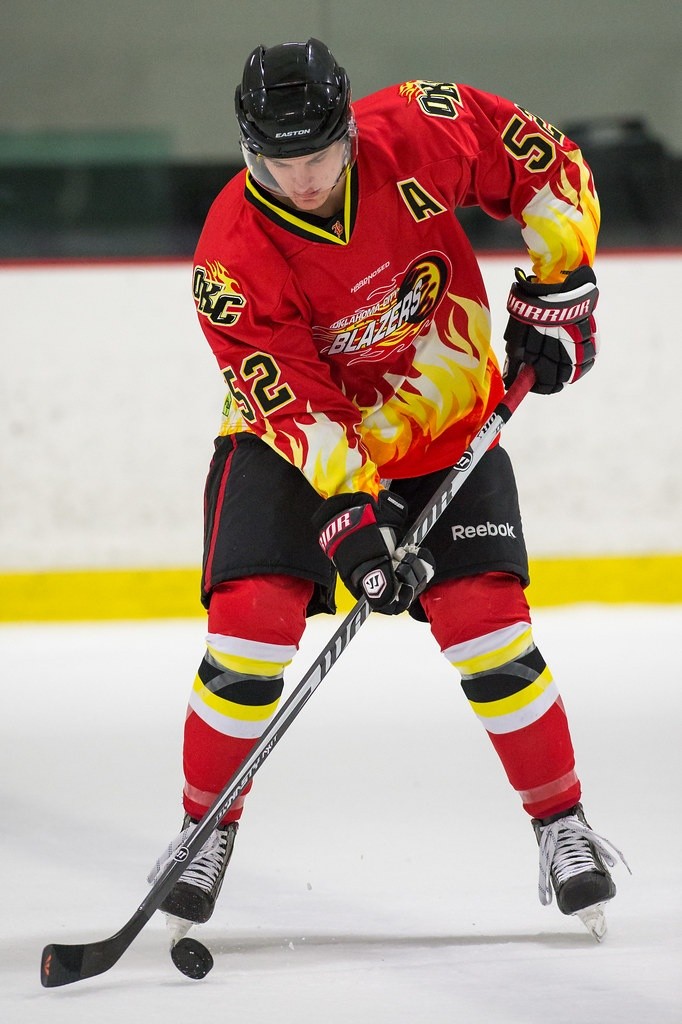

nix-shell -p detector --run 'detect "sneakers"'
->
[532,802,632,944]
[148,811,239,944]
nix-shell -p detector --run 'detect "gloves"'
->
[311,490,435,616]
[503,264,599,395]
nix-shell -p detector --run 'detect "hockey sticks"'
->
[42,363,540,990]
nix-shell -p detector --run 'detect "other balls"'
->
[170,938,214,980]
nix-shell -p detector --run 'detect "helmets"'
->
[235,38,359,176]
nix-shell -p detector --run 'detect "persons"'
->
[147,37,632,959]
